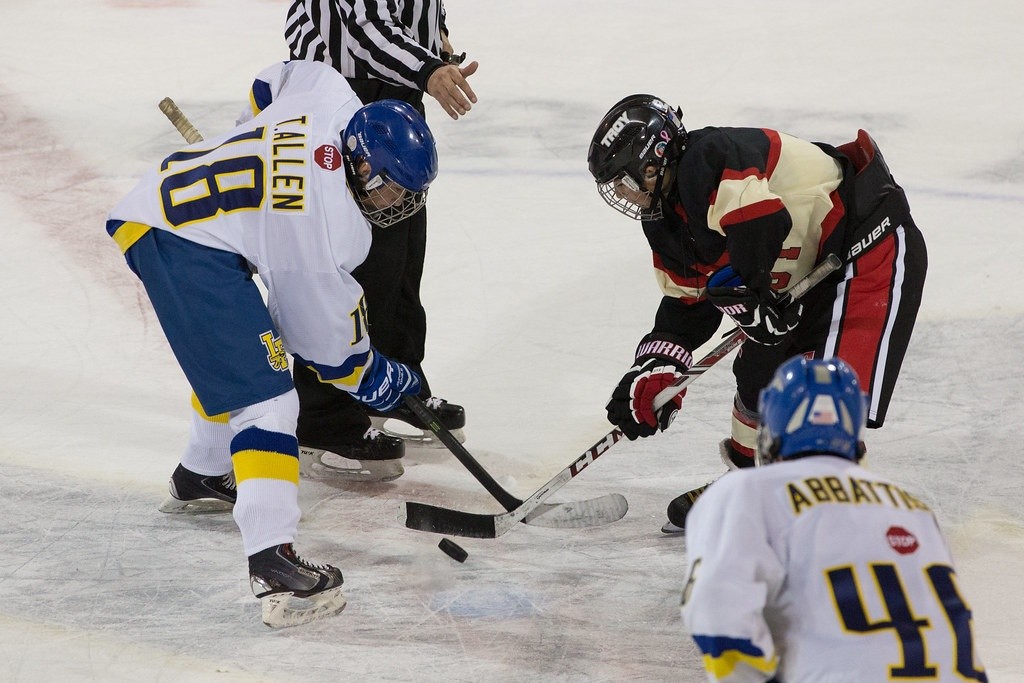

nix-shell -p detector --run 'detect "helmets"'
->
[342,100,438,228]
[588,94,688,222]
[754,354,867,467]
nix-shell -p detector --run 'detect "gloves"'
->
[347,345,421,413]
[705,264,803,346]
[606,332,693,441]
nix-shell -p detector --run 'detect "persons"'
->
[586,94,929,539]
[679,353,992,683]
[105,56,437,631]
[288,0,481,484]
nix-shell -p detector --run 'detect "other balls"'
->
[438,536,468,563]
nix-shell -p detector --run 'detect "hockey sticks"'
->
[400,253,844,541]
[157,94,629,529]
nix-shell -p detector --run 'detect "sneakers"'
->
[661,438,760,533]
[299,424,405,481]
[365,395,466,449]
[248,543,347,628]
[158,462,237,513]
